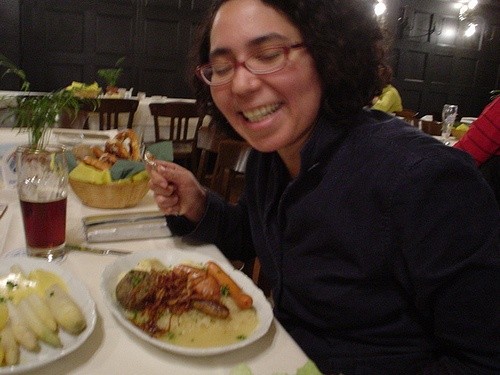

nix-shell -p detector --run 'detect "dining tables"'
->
[0,127,323,375]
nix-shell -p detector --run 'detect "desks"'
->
[0,91,211,140]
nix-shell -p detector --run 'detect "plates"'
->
[0,262,97,375]
[99,246,274,356]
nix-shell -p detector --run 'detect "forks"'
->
[137,124,167,177]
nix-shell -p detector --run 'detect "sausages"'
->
[206,261,253,308]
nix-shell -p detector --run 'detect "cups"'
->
[440,104,458,145]
[16,145,69,259]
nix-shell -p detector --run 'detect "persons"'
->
[368,63,402,113]
[145,0,500,375]
[189,112,245,273]
[452,94,500,204]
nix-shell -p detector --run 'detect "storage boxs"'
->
[422,121,442,136]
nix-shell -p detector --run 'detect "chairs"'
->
[210,140,264,285]
[150,101,207,172]
[82,98,139,131]
[392,109,419,127]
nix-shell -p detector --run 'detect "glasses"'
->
[194,40,314,87]
